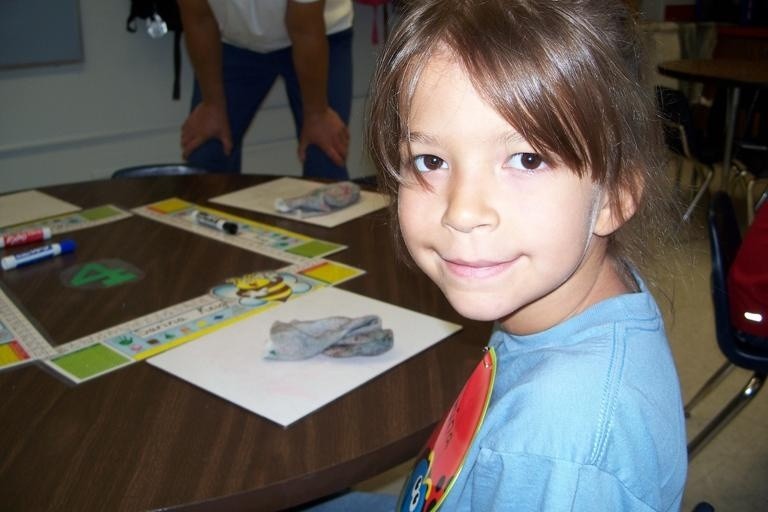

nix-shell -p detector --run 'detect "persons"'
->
[305,0,689,511]
[179,0,354,182]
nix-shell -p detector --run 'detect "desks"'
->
[2,173,494,509]
[656,35,768,228]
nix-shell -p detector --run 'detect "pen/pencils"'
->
[1,237,75,272]
[188,210,240,238]
[0,225,53,248]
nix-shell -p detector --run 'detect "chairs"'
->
[656,86,767,461]
